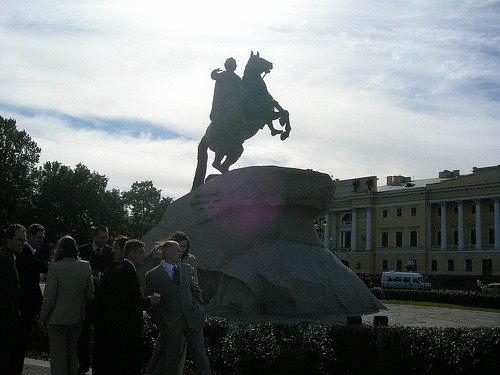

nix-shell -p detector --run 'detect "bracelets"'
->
[147,295,152,305]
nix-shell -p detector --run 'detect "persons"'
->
[210,58,283,136]
[13,223,49,375]
[145,230,197,375]
[355,272,373,288]
[93,236,129,286]
[76,226,114,375]
[37,235,95,375]
[0,223,26,375]
[143,240,212,375]
[104,239,160,375]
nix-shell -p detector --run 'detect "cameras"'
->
[97,272,105,282]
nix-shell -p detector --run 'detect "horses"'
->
[191,51,291,191]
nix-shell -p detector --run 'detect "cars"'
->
[481,283,500,296]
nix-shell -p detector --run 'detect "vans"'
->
[381,271,432,291]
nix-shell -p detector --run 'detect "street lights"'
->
[313,215,328,239]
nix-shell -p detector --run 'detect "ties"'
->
[96,248,101,260]
[172,266,180,284]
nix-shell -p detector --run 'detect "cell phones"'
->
[154,293,160,297]
